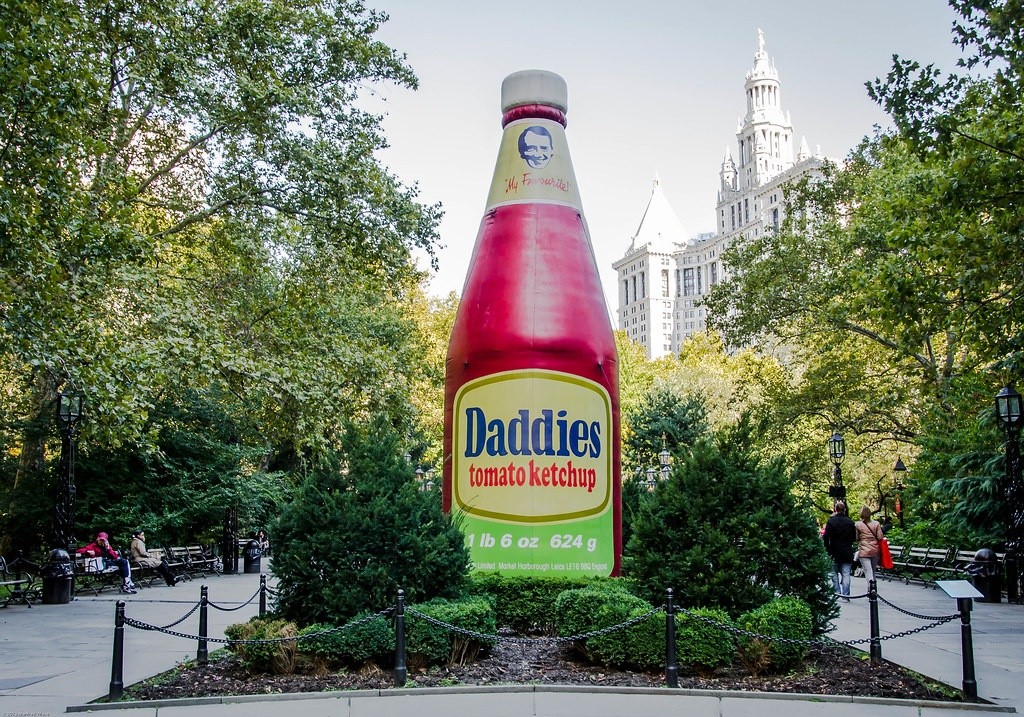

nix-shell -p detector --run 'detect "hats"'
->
[98,532,108,542]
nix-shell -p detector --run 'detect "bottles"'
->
[442,68,621,583]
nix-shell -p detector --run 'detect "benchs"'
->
[0,544,222,609]
[876,543,1006,590]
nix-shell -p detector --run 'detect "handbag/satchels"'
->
[84,557,103,572]
[877,538,893,568]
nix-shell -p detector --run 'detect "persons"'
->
[855,507,883,593]
[822,502,856,603]
[882,519,892,534]
[130,530,180,585]
[75,532,138,594]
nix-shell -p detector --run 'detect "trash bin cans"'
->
[41,549,72,604]
[244,541,262,573]
[973,548,1001,603]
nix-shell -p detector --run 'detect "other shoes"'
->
[167,577,181,587]
[123,580,137,594]
[842,598,850,603]
